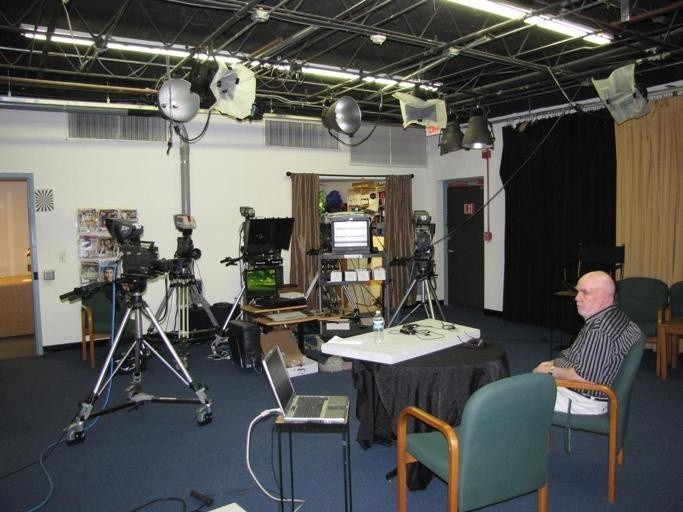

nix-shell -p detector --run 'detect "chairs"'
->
[395,371,556,512]
[613,276,669,376]
[660,280,683,379]
[547,330,646,504]
[81,282,138,369]
[551,241,625,346]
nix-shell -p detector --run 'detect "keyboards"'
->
[267,311,307,321]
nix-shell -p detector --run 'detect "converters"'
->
[191,490,213,506]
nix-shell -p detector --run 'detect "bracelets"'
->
[548,367,556,375]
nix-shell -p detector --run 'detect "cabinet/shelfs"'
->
[316,252,391,335]
[241,303,320,354]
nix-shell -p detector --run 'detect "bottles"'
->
[372,310,385,344]
[26,248,32,281]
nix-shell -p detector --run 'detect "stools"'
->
[274,402,353,512]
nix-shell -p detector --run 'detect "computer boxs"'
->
[228,320,259,368]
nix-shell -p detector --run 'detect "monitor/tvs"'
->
[245,268,283,297]
[330,217,370,254]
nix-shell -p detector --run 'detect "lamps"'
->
[461,99,494,150]
[440,103,467,155]
[154,78,201,123]
[318,94,362,137]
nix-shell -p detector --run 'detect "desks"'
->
[350,340,510,449]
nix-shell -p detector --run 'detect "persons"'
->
[533,271,642,415]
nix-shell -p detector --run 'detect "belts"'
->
[568,388,608,402]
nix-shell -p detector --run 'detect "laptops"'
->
[261,344,351,425]
